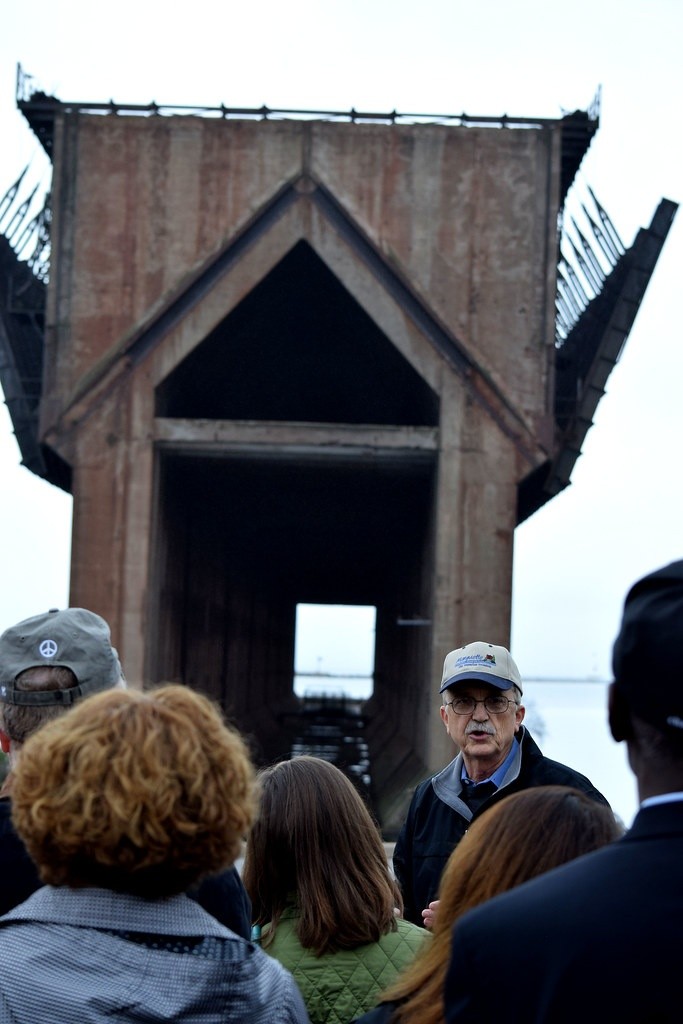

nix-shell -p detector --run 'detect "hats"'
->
[440,640,523,694]
[613,560,683,683]
[0,608,127,707]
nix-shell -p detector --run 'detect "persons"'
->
[350,787,623,1024]
[243,757,433,1024]
[393,641,616,932]
[0,608,253,941]
[0,686,310,1024]
[443,558,683,1024]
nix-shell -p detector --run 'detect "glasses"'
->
[446,694,517,715]
